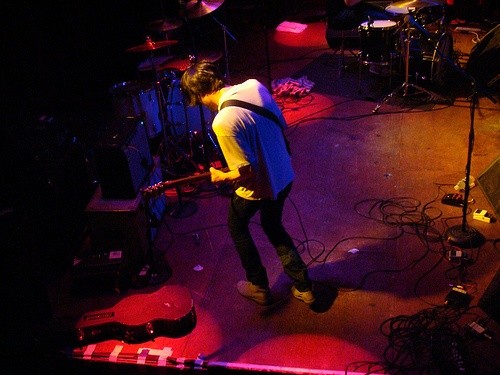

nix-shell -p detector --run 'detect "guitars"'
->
[138,168,230,207]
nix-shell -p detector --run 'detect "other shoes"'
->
[237,280,271,304]
[291,285,315,304]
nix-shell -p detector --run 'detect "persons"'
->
[325,0,396,76]
[179,59,316,305]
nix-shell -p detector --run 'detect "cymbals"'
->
[384,0,441,14]
[126,40,178,54]
[178,0,225,19]
[150,19,185,35]
[135,45,225,75]
[96,65,166,100]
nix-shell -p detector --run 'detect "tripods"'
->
[372,15,452,112]
[143,53,232,196]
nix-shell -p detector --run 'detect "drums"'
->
[385,30,454,92]
[358,19,403,64]
